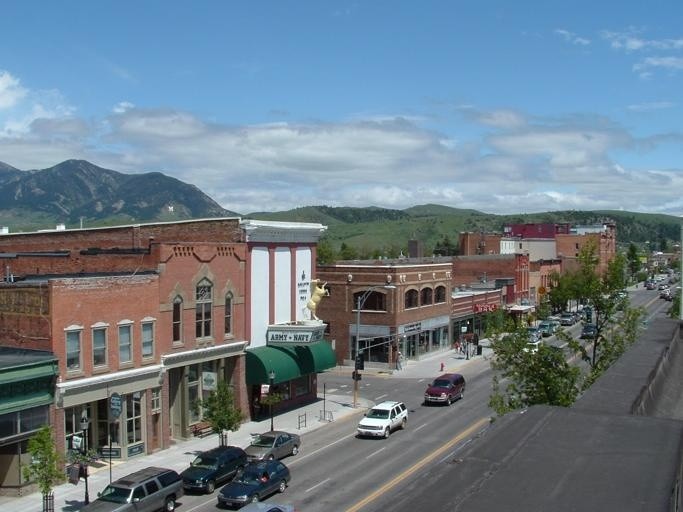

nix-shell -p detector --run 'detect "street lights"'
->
[351,284,397,406]
[79,418,90,504]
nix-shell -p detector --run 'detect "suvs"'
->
[423,373,465,407]
[80,467,182,512]
[179,445,246,495]
[357,400,409,440]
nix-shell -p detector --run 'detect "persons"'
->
[453,333,478,357]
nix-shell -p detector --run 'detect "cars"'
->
[217,459,291,507]
[580,325,599,338]
[517,310,584,343]
[245,428,302,463]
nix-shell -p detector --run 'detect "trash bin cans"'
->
[477,346,482,355]
